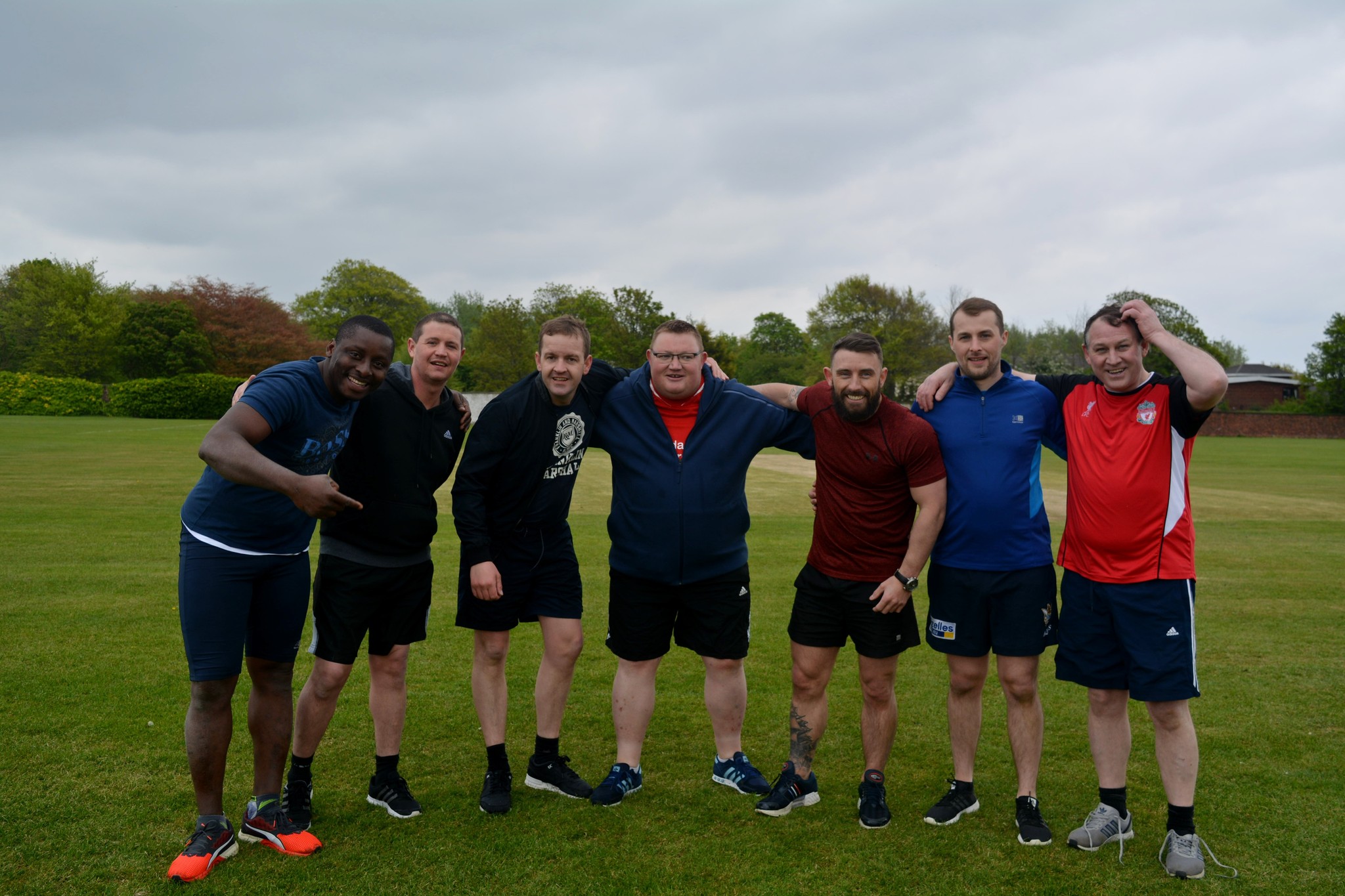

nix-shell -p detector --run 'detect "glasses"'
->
[650,348,705,364]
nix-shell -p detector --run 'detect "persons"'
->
[232,313,466,832]
[449,315,665,817]
[808,298,1068,845]
[703,334,950,832]
[587,321,816,812]
[165,317,472,887]
[913,299,1231,882]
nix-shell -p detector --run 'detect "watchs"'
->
[893,568,918,592]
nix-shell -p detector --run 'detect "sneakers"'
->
[282,780,313,831]
[524,754,593,799]
[366,775,422,819]
[924,773,979,825]
[593,763,643,807]
[756,766,821,816]
[169,817,239,885]
[1159,829,1237,879]
[1016,791,1052,846]
[857,769,891,828]
[1067,802,1134,866]
[712,752,771,796]
[479,767,514,814]
[237,794,323,856]
